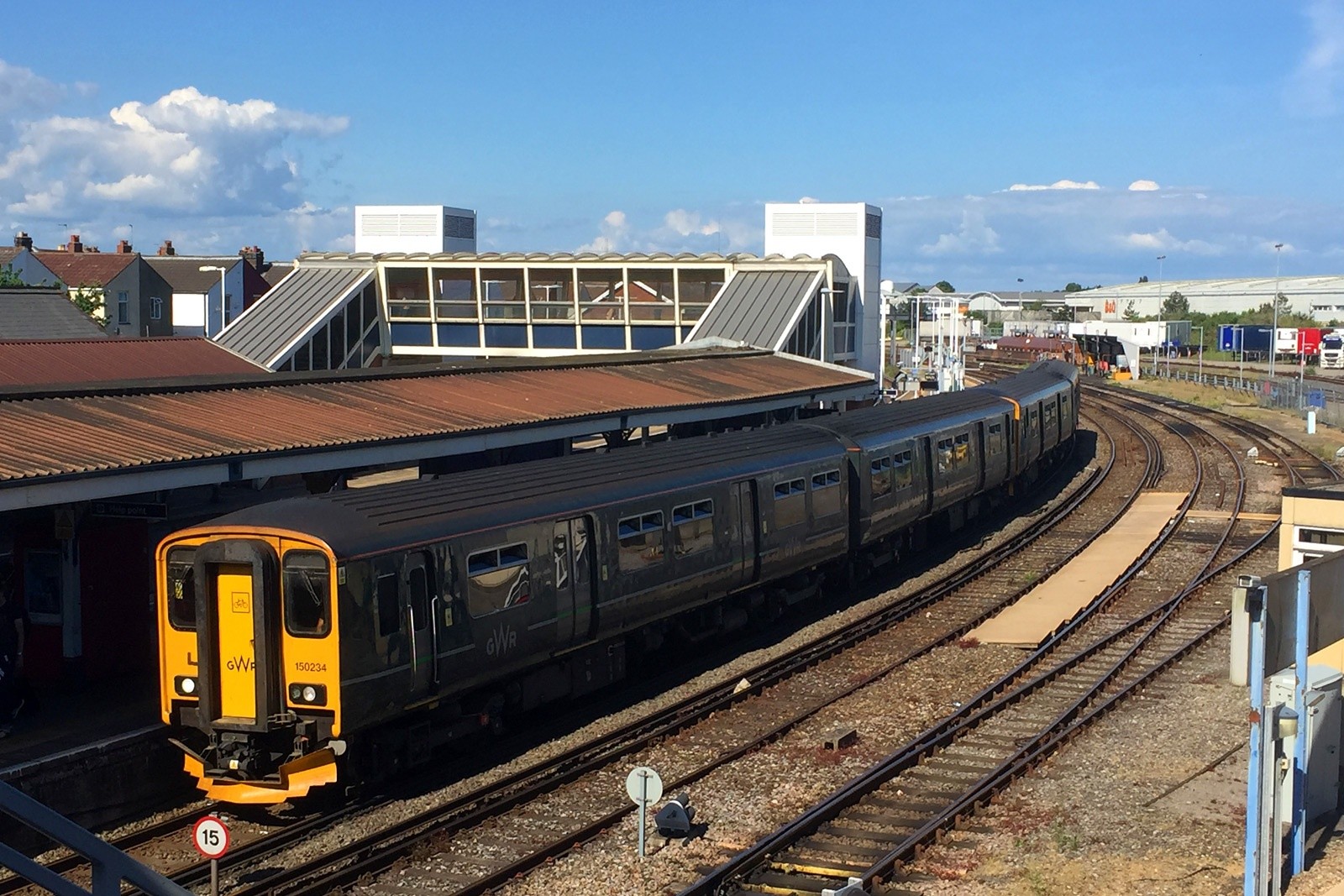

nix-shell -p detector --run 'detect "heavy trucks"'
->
[1318,331,1344,369]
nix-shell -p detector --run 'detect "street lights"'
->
[1231,327,1244,383]
[1271,243,1284,377]
[1156,254,1167,363]
[1290,331,1305,410]
[1191,326,1203,380]
[1159,325,1171,373]
[1259,328,1273,376]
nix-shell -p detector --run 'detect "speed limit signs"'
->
[192,817,230,859]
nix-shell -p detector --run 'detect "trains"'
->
[154,359,1080,807]
